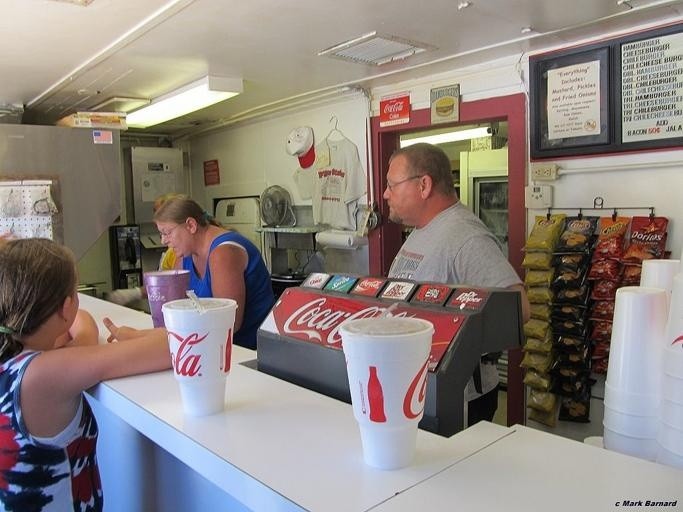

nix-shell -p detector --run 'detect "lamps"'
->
[399,120,499,149]
[125,75,242,129]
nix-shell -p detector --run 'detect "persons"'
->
[0,237,173,511]
[107,192,181,306]
[383,142,531,429]
[154,196,276,350]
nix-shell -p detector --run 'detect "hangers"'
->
[324,112,344,140]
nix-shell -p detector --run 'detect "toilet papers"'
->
[316,231,354,247]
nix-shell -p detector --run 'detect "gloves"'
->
[110,286,141,305]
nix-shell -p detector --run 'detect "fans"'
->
[259,184,293,229]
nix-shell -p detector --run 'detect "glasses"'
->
[387,174,422,192]
[160,224,180,238]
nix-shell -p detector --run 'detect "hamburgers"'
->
[435,97,455,117]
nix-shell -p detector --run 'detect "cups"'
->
[164,296,238,415]
[145,269,190,333]
[604,252,682,471]
[339,315,435,475]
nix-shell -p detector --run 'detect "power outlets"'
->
[530,162,560,182]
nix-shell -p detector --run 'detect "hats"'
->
[285,127,314,168]
